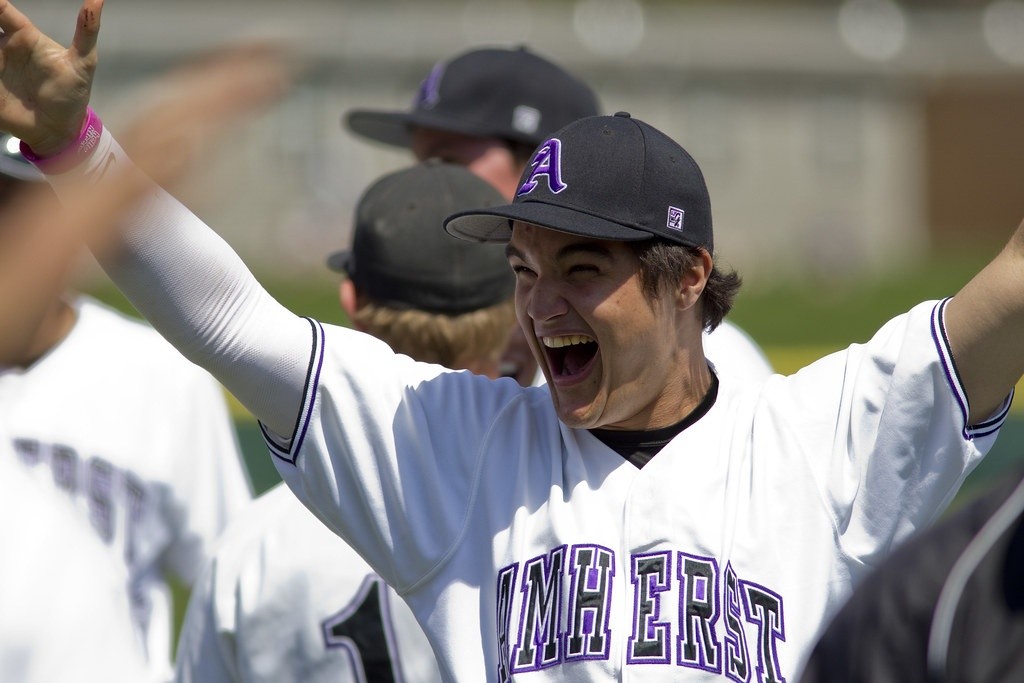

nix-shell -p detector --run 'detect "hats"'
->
[327,155,516,315]
[438,111,714,249]
[344,44,600,153]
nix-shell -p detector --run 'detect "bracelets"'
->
[20,108,103,174]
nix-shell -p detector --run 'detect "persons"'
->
[0,0,1024,683]
[801,470,1024,683]
[341,48,767,386]
[0,131,256,683]
[171,162,519,683]
